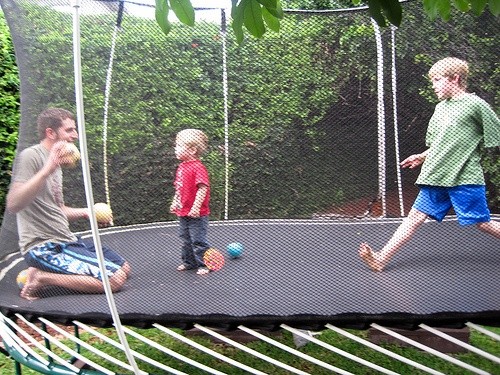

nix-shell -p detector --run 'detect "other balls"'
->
[60,144,79,164]
[227,242,242,258]
[94,203,112,223]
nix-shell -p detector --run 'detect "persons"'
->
[168,129,211,275]
[7,108,131,299]
[357,56,500,272]
